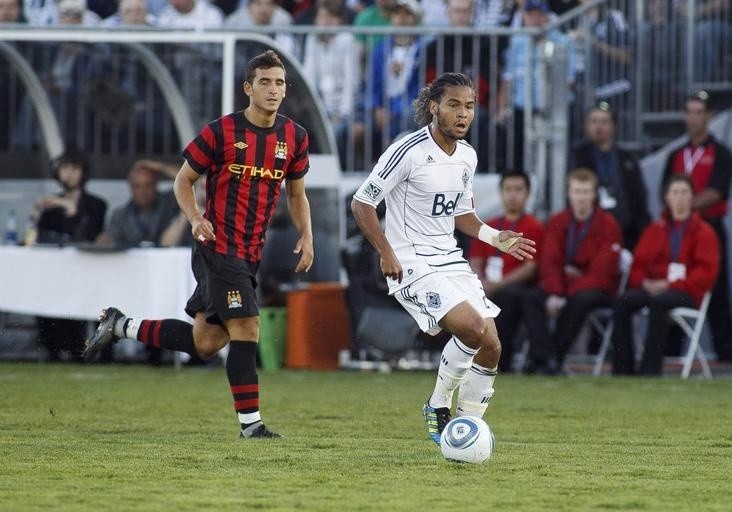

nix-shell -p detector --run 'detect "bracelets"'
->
[478,224,502,247]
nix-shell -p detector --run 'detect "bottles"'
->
[24,216,37,247]
[5,211,17,245]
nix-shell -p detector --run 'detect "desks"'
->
[0,243,229,369]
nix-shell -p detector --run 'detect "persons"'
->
[1,0,732,174]
[96,159,194,247]
[465,173,543,372]
[661,96,732,362]
[35,152,106,363]
[523,167,623,373]
[572,108,652,251]
[615,176,723,375]
[81,49,315,438]
[351,72,537,446]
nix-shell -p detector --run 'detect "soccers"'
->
[440,416,495,465]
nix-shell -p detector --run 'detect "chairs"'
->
[582,254,632,376]
[641,289,712,381]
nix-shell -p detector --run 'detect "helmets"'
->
[50,150,90,181]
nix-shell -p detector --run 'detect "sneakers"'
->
[240,424,280,438]
[81,307,124,360]
[423,400,451,446]
